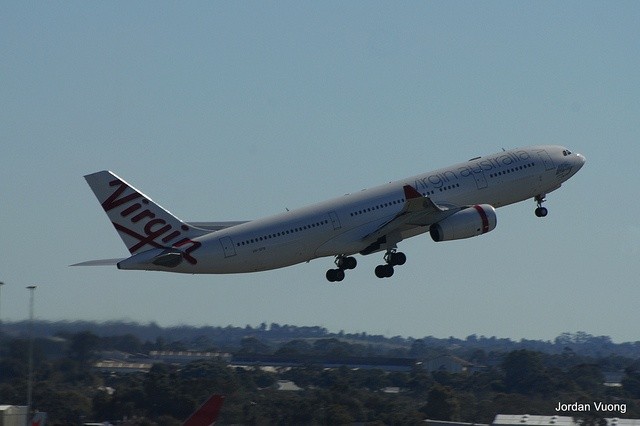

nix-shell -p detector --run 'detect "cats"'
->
[68,145,586,281]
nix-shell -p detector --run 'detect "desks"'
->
[26,285,36,425]
